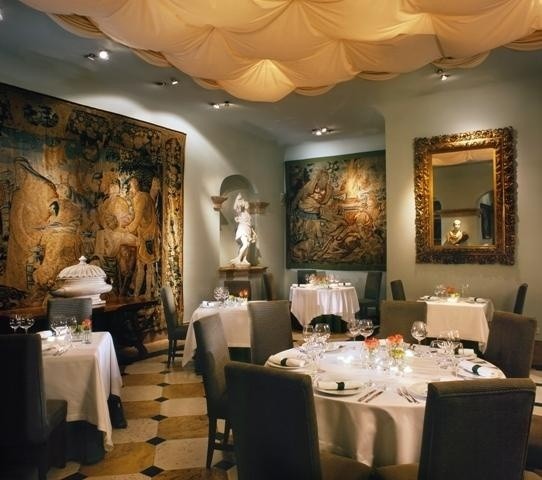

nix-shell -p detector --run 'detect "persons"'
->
[5,157,157,298]
[444,219,469,247]
[290,169,347,261]
[230,193,258,266]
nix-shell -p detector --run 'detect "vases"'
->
[361,344,381,370]
[450,294,455,300]
[387,343,406,375]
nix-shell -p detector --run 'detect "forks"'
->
[399,387,418,403]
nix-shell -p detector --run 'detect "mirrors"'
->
[476,190,493,209]
[412,124,517,266]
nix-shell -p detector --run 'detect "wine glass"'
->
[329,272,341,287]
[213,287,229,307]
[10,313,21,335]
[434,285,446,300]
[302,323,331,374]
[411,321,428,345]
[349,320,360,345]
[50,315,77,339]
[20,312,34,335]
[431,329,465,378]
[358,319,374,343]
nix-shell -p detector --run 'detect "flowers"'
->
[448,286,456,293]
[363,338,379,364]
[385,333,404,354]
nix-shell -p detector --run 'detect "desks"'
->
[0,294,159,371]
[219,265,267,299]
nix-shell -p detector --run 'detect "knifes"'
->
[358,389,384,402]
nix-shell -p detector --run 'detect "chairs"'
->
[374,301,427,345]
[1,333,67,479]
[486,310,537,380]
[297,269,317,286]
[160,286,189,369]
[223,359,371,480]
[190,313,239,469]
[390,280,406,301]
[248,299,293,365]
[376,379,535,480]
[513,283,528,315]
[358,271,382,316]
[47,298,93,333]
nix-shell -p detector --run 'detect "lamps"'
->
[312,127,329,137]
[84,53,95,60]
[209,102,219,111]
[221,102,231,112]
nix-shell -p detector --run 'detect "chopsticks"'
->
[474,361,487,363]
[463,368,473,374]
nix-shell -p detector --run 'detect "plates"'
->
[268,361,301,369]
[317,387,362,396]
[477,366,502,378]
